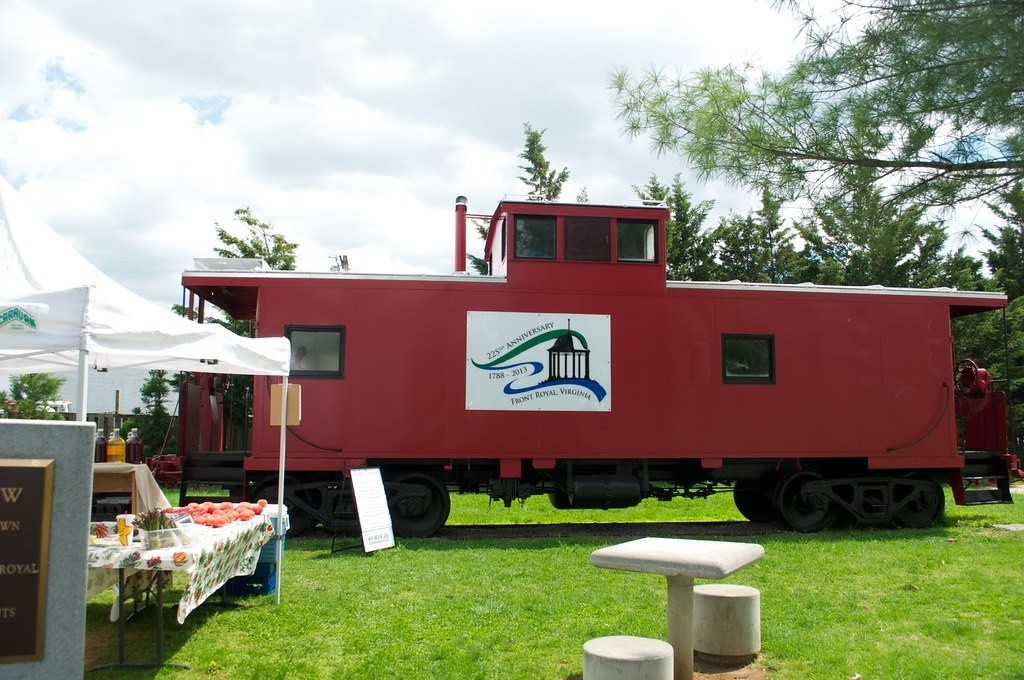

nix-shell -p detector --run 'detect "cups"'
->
[116,514,135,546]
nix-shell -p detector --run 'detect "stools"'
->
[582,636,675,680]
[693,584,761,656]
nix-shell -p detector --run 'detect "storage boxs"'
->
[200,502,289,597]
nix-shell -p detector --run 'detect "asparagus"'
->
[132,508,191,549]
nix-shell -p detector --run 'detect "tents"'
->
[1,174,292,605]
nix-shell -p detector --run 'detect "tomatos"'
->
[165,499,267,527]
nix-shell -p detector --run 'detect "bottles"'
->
[94,428,108,462]
[126,428,143,463]
[107,428,126,463]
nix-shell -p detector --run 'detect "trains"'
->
[150,195,1024,541]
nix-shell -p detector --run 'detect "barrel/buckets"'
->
[214,503,291,597]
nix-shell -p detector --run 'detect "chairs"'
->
[327,490,370,556]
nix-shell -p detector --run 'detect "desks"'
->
[590,536,765,680]
[93,462,172,517]
[87,513,275,670]
